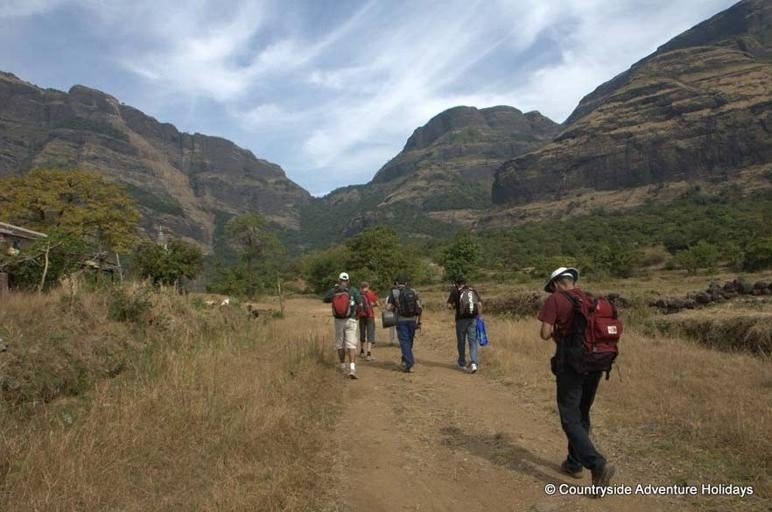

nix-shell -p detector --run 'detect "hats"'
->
[338,272,349,281]
[543,266,580,293]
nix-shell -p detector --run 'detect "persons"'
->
[447,276,484,374]
[355,281,381,362]
[384,275,424,373]
[537,265,617,499]
[322,272,363,379]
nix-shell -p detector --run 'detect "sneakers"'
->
[560,454,584,479]
[589,462,616,500]
[457,363,479,375]
[340,367,361,379]
[359,352,376,362]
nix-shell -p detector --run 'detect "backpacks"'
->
[391,285,417,318]
[331,287,356,320]
[559,290,623,380]
[356,293,373,320]
[457,287,479,320]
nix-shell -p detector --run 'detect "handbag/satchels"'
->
[476,317,488,346]
